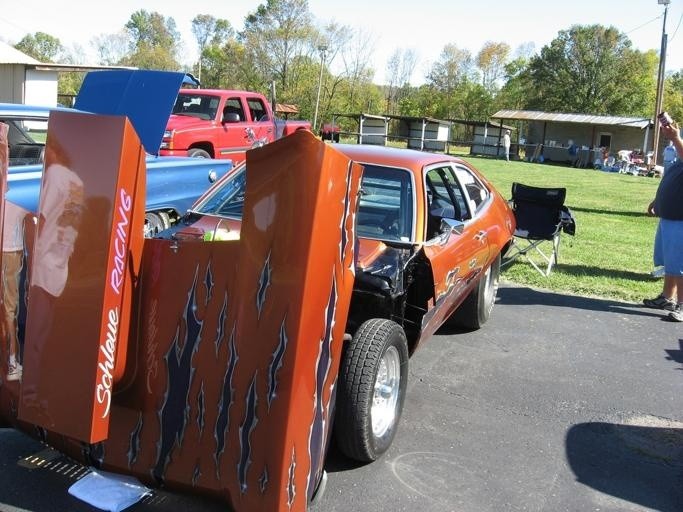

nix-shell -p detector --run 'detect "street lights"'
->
[313,44,329,131]
[652,0,671,168]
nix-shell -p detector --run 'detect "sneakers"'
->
[643,293,683,322]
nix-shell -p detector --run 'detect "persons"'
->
[1,197,36,384]
[502,130,512,161]
[564,138,679,177]
[642,123,683,323]
[13,134,85,406]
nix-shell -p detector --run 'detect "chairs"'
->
[501,181,566,279]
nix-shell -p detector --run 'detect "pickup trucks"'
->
[159,89,313,167]
